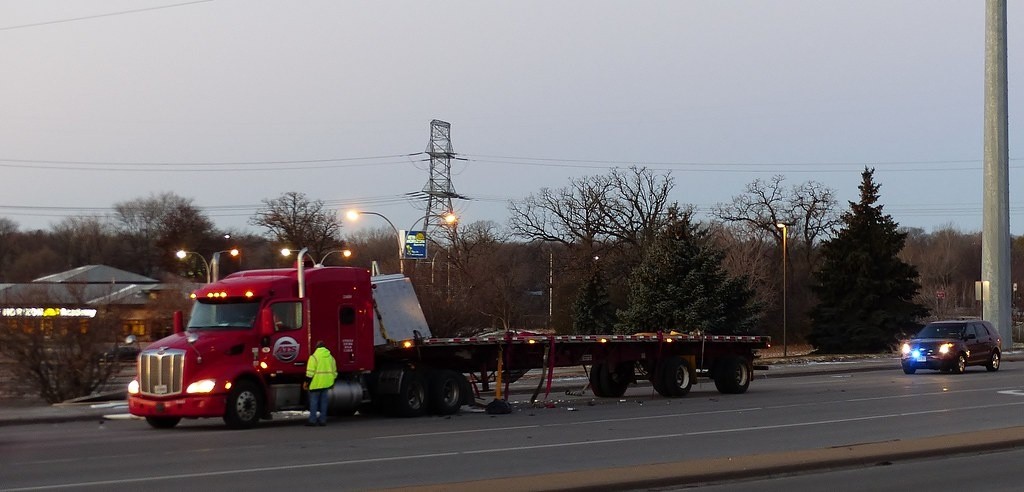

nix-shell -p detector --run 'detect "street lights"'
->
[176,248,239,282]
[281,247,352,268]
[777,223,787,357]
[431,245,454,291]
[549,256,600,326]
[347,210,457,274]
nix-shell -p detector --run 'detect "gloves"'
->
[303,381,309,390]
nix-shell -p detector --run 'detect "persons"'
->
[253,308,283,328]
[304,341,338,427]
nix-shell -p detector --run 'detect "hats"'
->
[316,342,325,347]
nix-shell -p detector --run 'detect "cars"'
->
[101,345,141,362]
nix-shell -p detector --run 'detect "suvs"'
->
[901,318,1002,374]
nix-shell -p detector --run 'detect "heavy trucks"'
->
[128,246,772,431]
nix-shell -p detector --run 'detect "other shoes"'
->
[303,421,316,427]
[315,420,326,427]
